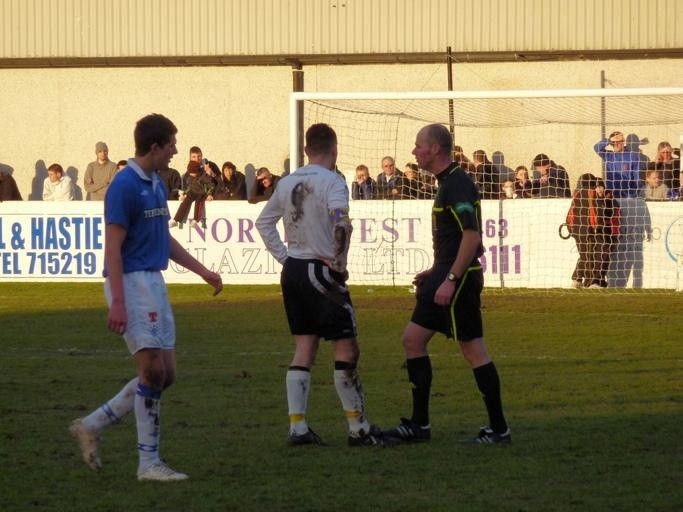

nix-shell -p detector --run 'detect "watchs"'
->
[447,271,459,283]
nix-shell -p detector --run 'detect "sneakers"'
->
[383,416,431,442]
[69,418,101,472]
[287,427,328,445]
[138,462,188,481]
[460,426,513,445]
[349,424,400,447]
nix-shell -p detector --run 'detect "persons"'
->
[42,164,76,199]
[567,174,605,289]
[117,160,128,170]
[454,146,571,198]
[1,172,20,201]
[254,123,402,449]
[162,146,282,204]
[336,156,439,199]
[591,176,622,287]
[593,132,683,200]
[84,141,118,200]
[64,110,224,484]
[168,160,212,228]
[375,123,513,448]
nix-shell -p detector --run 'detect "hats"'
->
[95,142,107,154]
[188,162,200,173]
